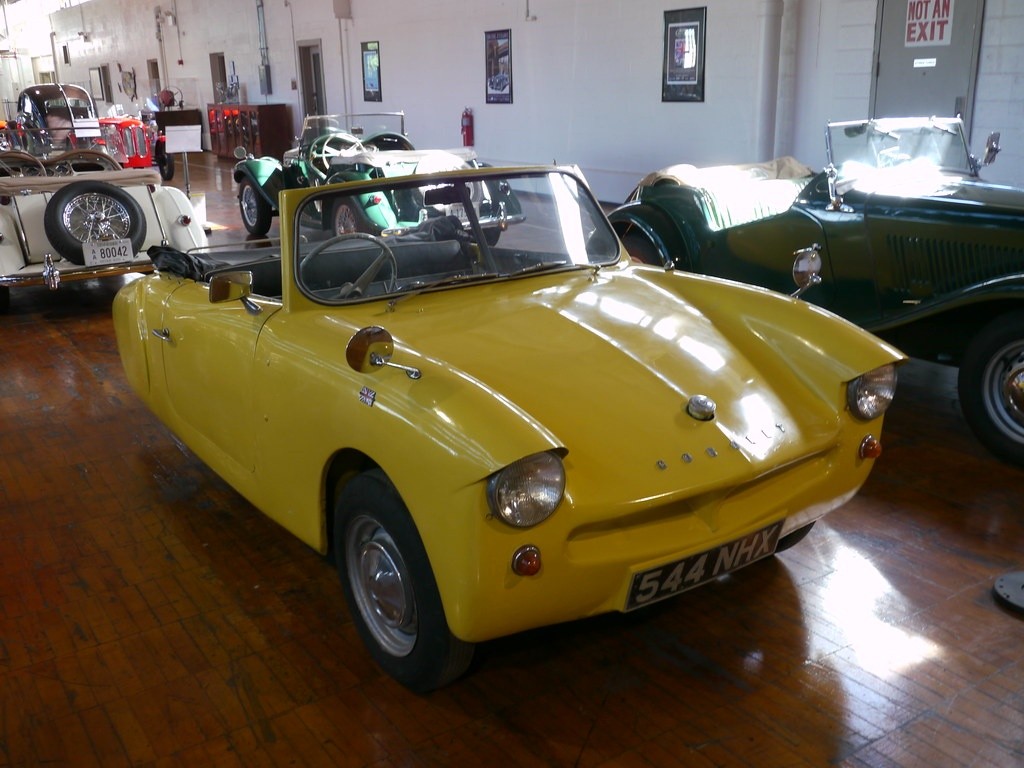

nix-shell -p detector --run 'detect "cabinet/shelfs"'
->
[207,102,288,162]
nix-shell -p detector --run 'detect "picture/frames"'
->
[360,41,382,102]
[659,6,707,102]
[485,28,513,104]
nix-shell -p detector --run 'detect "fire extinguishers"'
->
[461,106,474,147]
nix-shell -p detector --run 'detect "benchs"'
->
[204,240,470,296]
[0,169,163,196]
[682,175,814,233]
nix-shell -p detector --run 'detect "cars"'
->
[0,124,210,312]
[236,110,527,256]
[15,86,177,184]
[111,162,909,693]
[580,114,1023,464]
